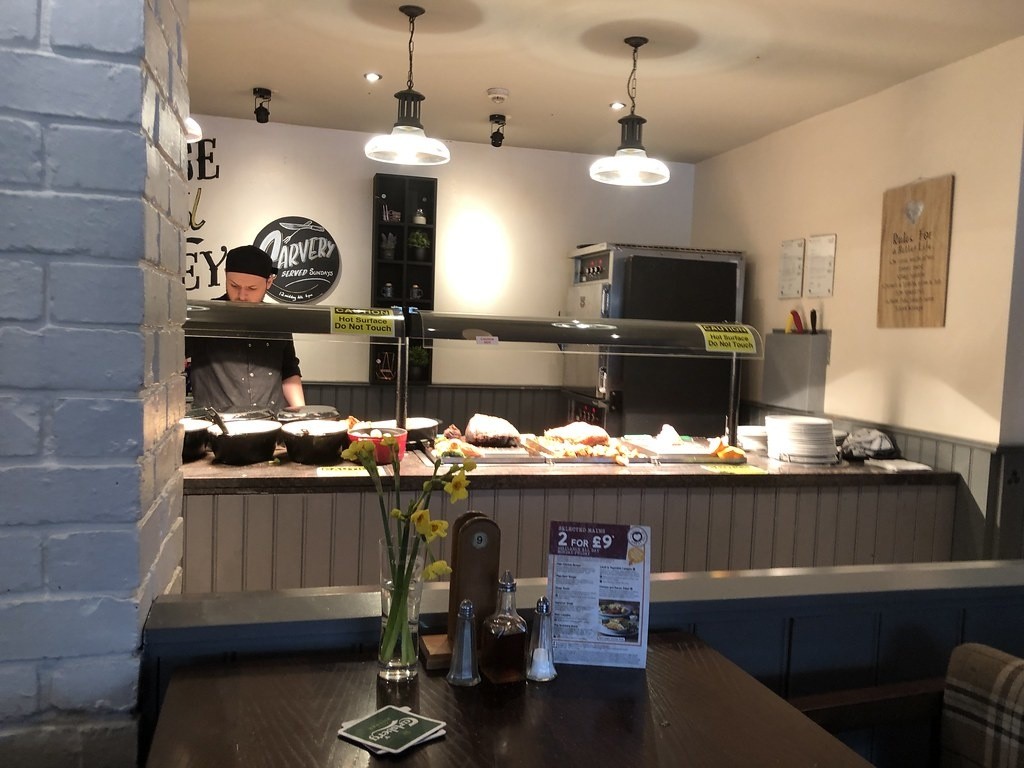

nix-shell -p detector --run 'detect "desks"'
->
[147,627,882,768]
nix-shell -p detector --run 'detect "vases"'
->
[378,536,431,682]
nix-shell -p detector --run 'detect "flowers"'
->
[342,426,480,584]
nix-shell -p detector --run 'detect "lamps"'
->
[364,6,450,167]
[589,37,671,189]
[252,88,271,123]
[490,114,506,148]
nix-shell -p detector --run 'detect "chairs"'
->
[939,642,1024,768]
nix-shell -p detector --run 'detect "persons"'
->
[186,247,307,423]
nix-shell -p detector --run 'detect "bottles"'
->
[384,282,393,297]
[527,596,557,681]
[446,599,481,687]
[481,568,527,669]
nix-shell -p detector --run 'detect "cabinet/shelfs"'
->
[367,174,438,386]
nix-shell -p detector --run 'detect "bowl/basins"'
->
[183,406,442,459]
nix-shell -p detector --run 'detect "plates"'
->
[726,414,838,464]
[599,607,630,616]
[601,623,638,635]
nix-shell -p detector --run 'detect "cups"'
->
[414,217,426,224]
[410,284,423,299]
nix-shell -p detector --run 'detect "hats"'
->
[225,244,278,278]
